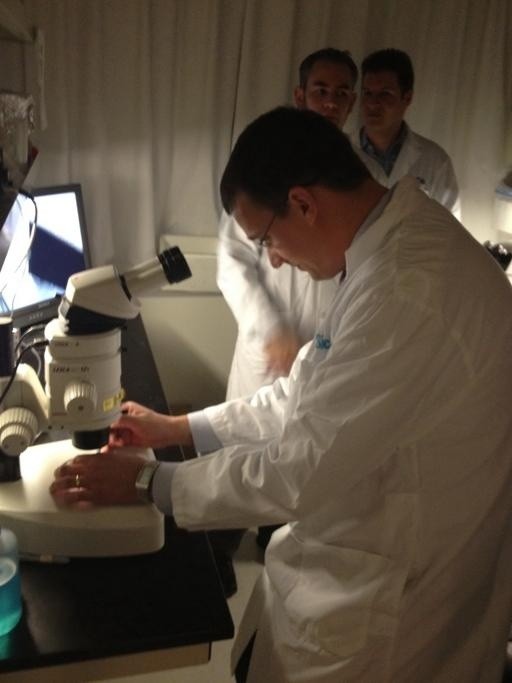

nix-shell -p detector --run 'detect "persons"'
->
[347,47,462,225]
[48,100,512,683]
[214,47,389,564]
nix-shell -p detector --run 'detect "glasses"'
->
[256,203,284,246]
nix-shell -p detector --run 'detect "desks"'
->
[0,312,239,680]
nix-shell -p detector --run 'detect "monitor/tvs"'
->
[0,182,93,327]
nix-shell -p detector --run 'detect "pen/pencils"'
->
[315,334,333,349]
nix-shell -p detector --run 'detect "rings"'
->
[76,473,80,487]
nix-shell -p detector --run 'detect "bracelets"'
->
[134,460,161,502]
